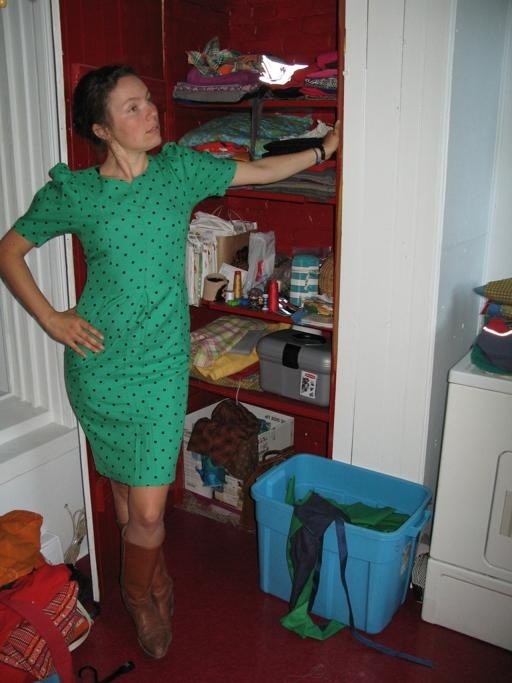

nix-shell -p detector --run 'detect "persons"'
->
[1,64,341,659]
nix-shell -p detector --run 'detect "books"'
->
[186,232,218,310]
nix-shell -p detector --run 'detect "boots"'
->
[117,521,177,657]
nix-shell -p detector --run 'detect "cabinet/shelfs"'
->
[49,3,364,525]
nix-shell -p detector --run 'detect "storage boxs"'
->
[243,450,436,640]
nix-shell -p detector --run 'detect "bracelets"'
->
[313,143,327,165]
